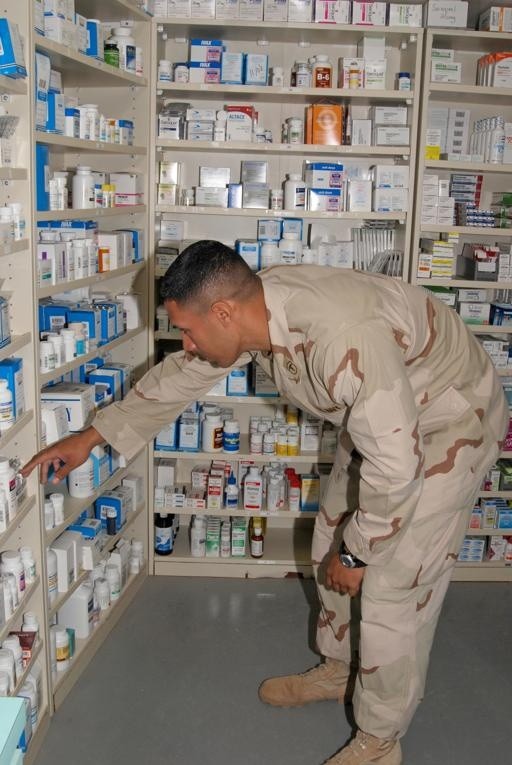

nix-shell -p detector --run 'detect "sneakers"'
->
[258,659,351,707]
[324,734,402,765]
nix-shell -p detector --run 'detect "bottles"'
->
[77,102,120,145]
[103,25,145,77]
[214,109,306,144]
[272,54,412,91]
[44,455,146,673]
[261,173,307,269]
[223,460,301,513]
[153,513,267,559]
[156,60,189,82]
[38,321,89,374]
[199,407,337,455]
[0,379,42,725]
[179,187,194,207]
[471,114,506,165]
[0,165,116,284]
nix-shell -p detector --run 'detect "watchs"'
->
[339,540,368,569]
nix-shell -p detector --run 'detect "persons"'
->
[19,240,510,765]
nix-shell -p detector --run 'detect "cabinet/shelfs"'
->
[0,0,159,765]
[157,17,511,582]
